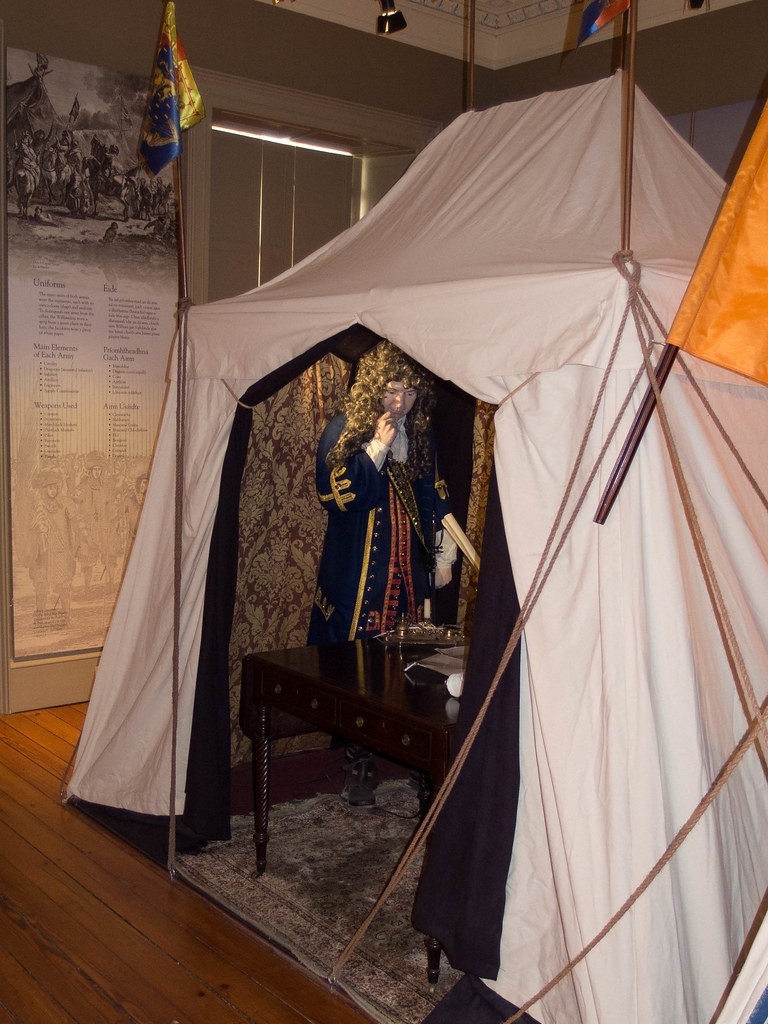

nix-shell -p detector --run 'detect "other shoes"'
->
[339,786,377,805]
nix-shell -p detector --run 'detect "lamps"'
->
[377,0,408,34]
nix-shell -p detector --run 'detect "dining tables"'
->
[238,637,462,985]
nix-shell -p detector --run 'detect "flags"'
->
[575,1,631,56]
[132,0,210,178]
[661,85,768,392]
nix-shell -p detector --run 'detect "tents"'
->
[58,60,767,1024]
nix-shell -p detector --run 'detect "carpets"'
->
[175,779,465,1024]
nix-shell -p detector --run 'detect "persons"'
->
[312,336,465,813]
